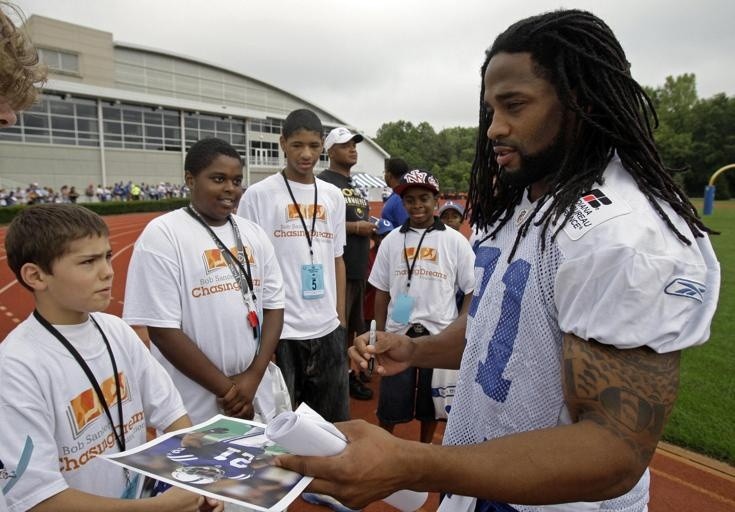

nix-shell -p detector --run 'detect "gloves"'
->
[208,428,229,434]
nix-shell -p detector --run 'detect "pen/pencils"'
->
[368,320,377,376]
[372,221,378,231]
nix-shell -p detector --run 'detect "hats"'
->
[385,158,440,199]
[369,216,395,235]
[439,200,466,218]
[324,127,364,152]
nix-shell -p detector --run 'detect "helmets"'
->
[172,465,225,485]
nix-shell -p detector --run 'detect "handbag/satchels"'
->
[430,368,460,422]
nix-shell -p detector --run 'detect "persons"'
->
[120,134,289,443]
[381,185,391,207]
[377,157,412,231]
[362,217,395,383]
[362,184,372,211]
[438,198,467,315]
[231,106,354,426]
[0,201,226,511]
[165,423,277,487]
[0,180,193,209]
[259,5,723,512]
[312,124,380,404]
[365,166,478,447]
[0,0,50,134]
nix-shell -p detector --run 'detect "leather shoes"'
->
[349,371,373,400]
